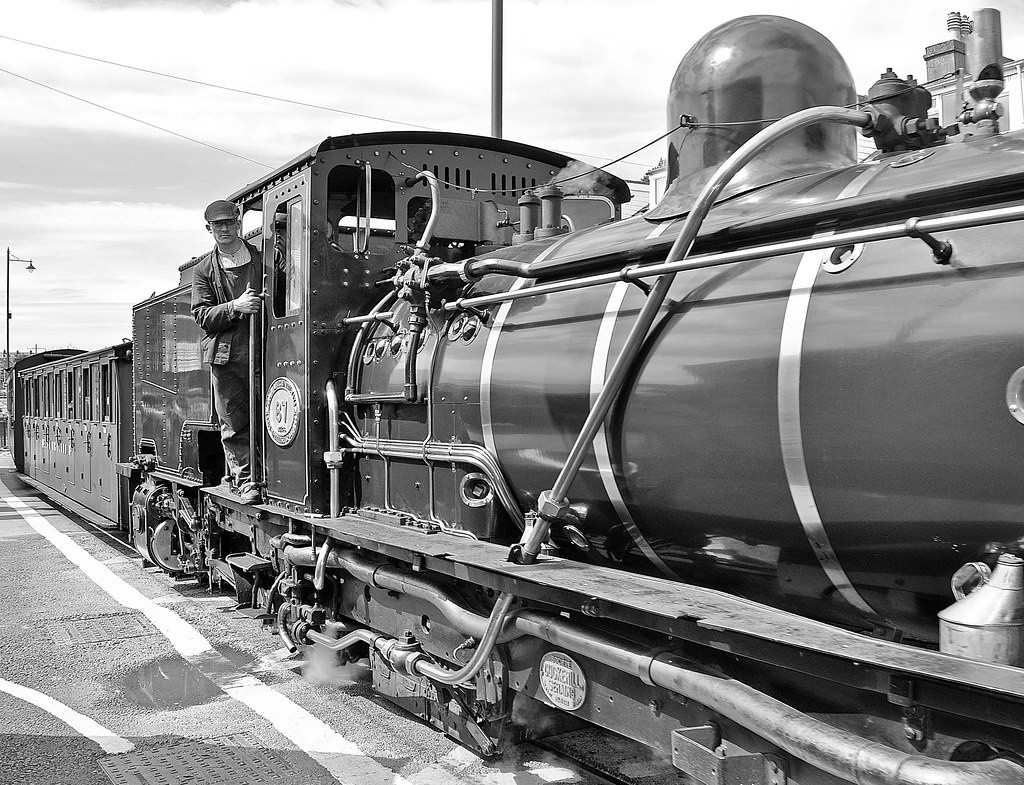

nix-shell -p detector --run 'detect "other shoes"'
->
[240,484,262,502]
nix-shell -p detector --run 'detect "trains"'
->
[7,8,1024,785]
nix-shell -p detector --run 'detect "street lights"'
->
[6,259,37,371]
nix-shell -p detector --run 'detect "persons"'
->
[190,200,285,507]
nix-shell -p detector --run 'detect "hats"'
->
[205,200,240,221]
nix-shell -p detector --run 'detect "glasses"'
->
[209,217,238,227]
